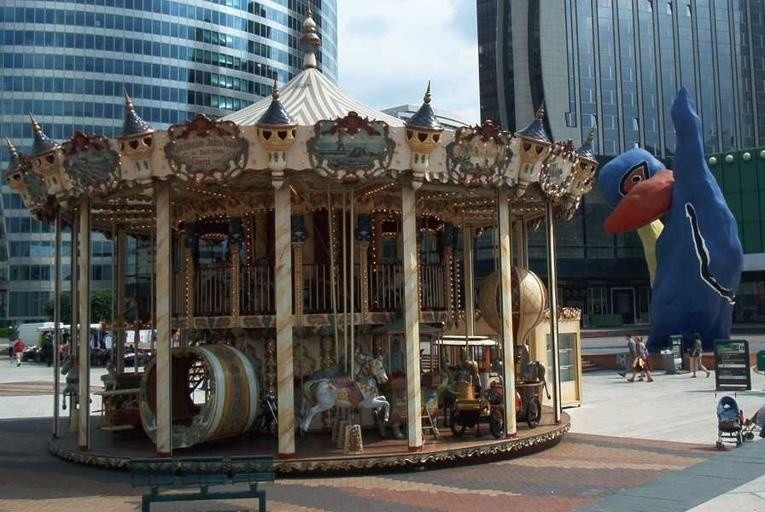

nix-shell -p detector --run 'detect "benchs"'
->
[129,453,276,512]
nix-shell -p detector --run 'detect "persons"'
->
[689,334,710,378]
[627,336,654,382]
[14,337,27,366]
[618,334,646,377]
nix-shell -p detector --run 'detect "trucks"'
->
[6,323,63,358]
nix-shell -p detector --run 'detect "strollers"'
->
[714,389,746,451]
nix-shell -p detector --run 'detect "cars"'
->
[21,343,157,369]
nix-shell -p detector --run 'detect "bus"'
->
[36,320,103,351]
